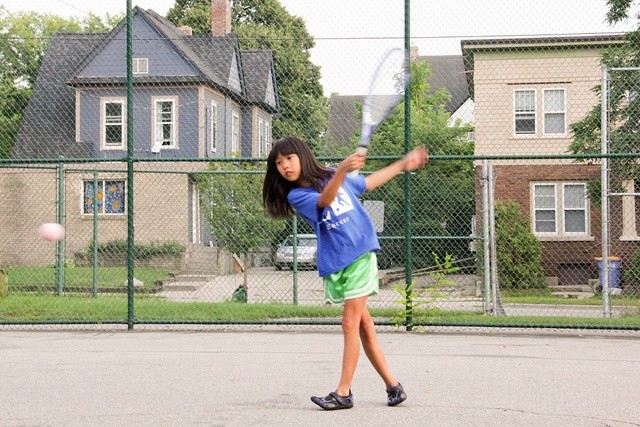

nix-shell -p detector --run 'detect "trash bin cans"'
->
[594,255,622,287]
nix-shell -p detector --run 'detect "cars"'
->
[273,233,317,270]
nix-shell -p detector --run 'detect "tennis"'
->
[39,223,65,241]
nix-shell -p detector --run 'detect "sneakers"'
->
[311,392,354,409]
[386,382,407,406]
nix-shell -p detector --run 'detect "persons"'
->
[263,137,430,409]
